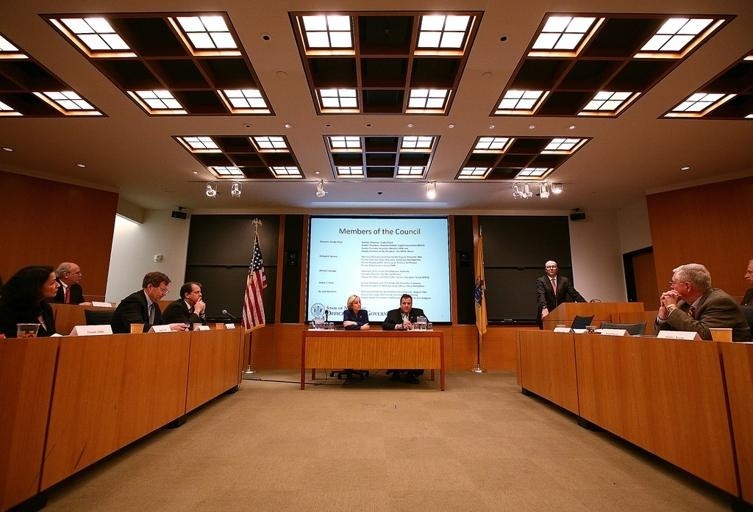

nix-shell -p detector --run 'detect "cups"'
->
[192,323,201,329]
[312,322,335,330]
[585,326,595,334]
[410,322,433,332]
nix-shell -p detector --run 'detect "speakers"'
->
[570,213,586,220]
[171,211,186,220]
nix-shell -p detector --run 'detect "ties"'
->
[149,304,153,322]
[687,306,696,317]
[66,287,71,304]
[551,278,558,296]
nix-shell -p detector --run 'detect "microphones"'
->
[324,309,329,318]
[221,309,238,323]
[409,312,414,322]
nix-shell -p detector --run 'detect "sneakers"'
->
[398,373,419,385]
[342,373,369,385]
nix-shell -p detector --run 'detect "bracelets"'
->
[200,313,205,315]
[666,304,676,310]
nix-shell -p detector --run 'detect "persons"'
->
[653,263,753,342]
[45,262,92,306]
[382,294,430,384]
[343,295,371,330]
[740,259,753,306]
[111,272,187,334]
[161,281,206,330]
[0,264,61,338]
[535,260,587,330]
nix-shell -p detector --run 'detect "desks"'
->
[574,331,738,497]
[0,336,59,511]
[40,331,192,493]
[186,326,245,420]
[716,340,751,510]
[301,329,445,391]
[515,328,579,415]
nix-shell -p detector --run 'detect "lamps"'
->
[227,180,242,198]
[424,178,437,200]
[507,181,563,199]
[203,179,221,200]
[314,178,326,197]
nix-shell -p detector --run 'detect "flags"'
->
[241,234,268,335]
[474,236,488,337]
